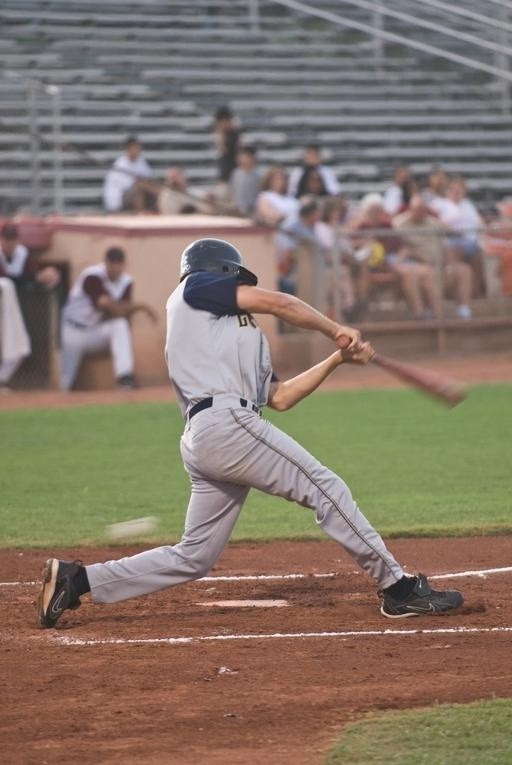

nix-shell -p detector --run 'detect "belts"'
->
[189,396,263,422]
[65,317,88,330]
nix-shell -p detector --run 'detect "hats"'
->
[106,247,125,262]
[1,221,20,240]
[179,237,258,289]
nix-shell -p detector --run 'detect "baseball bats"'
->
[334,335,467,407]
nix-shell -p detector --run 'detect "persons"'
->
[58,247,159,390]
[227,144,512,318]
[103,134,195,215]
[215,107,240,183]
[0,222,40,282]
[38,238,465,628]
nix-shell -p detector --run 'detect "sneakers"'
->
[380,571,466,619]
[117,373,137,391]
[36,557,84,630]
